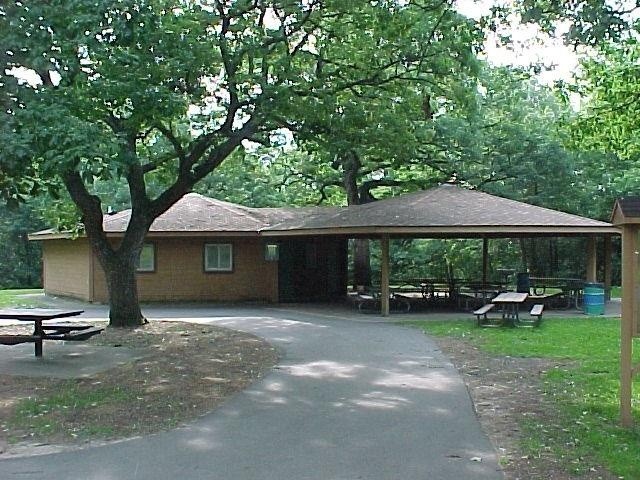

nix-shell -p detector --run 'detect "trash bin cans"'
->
[517,272,530,295]
[583,281,605,316]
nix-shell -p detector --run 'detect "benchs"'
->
[350,280,585,314]
[0,325,104,343]
[472,303,545,317]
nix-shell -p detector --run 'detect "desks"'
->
[490,292,530,328]
[0,308,83,358]
